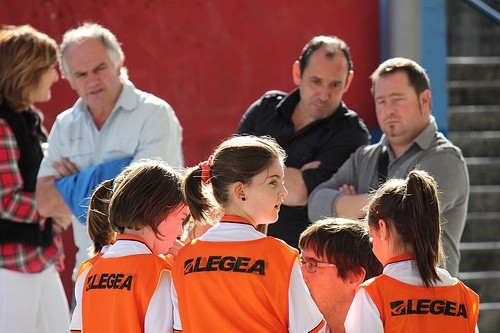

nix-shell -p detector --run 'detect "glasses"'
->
[299,258,336,273]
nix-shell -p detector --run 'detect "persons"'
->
[307,58,470,279]
[70,135,482,333]
[0,24,69,333]
[233,35,370,252]
[35,22,186,333]
